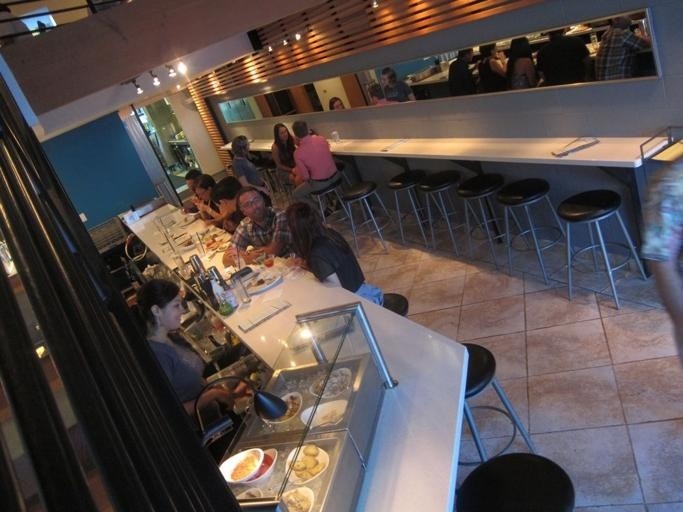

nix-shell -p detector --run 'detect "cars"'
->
[498,51,505,65]
[171,252,188,276]
[231,276,250,304]
[590,33,600,50]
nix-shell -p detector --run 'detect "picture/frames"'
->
[217,135,673,280]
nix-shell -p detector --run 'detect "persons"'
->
[505,37,544,90]
[327,96,345,112]
[446,47,479,96]
[230,134,272,206]
[270,121,299,189]
[366,83,400,107]
[290,118,341,217]
[285,202,383,306]
[638,155,682,367]
[179,169,272,236]
[381,67,416,103]
[535,27,591,87]
[219,185,293,270]
[592,15,645,81]
[476,42,509,93]
[129,278,234,462]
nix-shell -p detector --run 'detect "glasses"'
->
[129,55,178,96]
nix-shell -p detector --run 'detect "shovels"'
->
[289,445,326,482]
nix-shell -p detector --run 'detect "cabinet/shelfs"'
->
[188,253,239,314]
[331,129,341,142]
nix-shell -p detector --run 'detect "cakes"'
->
[331,131,340,142]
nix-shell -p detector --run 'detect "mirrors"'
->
[216,6,664,127]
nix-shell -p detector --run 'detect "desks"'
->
[220,135,675,235]
[121,196,469,512]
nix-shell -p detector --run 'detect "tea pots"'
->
[216,6,664,127]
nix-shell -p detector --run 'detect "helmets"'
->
[154,211,232,250]
[240,269,282,296]
[218,392,350,511]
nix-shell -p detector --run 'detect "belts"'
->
[230,246,300,271]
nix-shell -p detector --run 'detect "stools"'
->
[500,174,577,285]
[556,188,648,310]
[246,149,359,232]
[374,293,410,317]
[453,452,577,508]
[390,168,445,247]
[313,169,338,182]
[344,180,395,252]
[459,171,529,269]
[421,166,478,256]
[457,342,535,469]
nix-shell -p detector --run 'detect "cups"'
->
[242,197,263,211]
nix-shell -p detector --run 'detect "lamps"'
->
[129,55,178,96]
